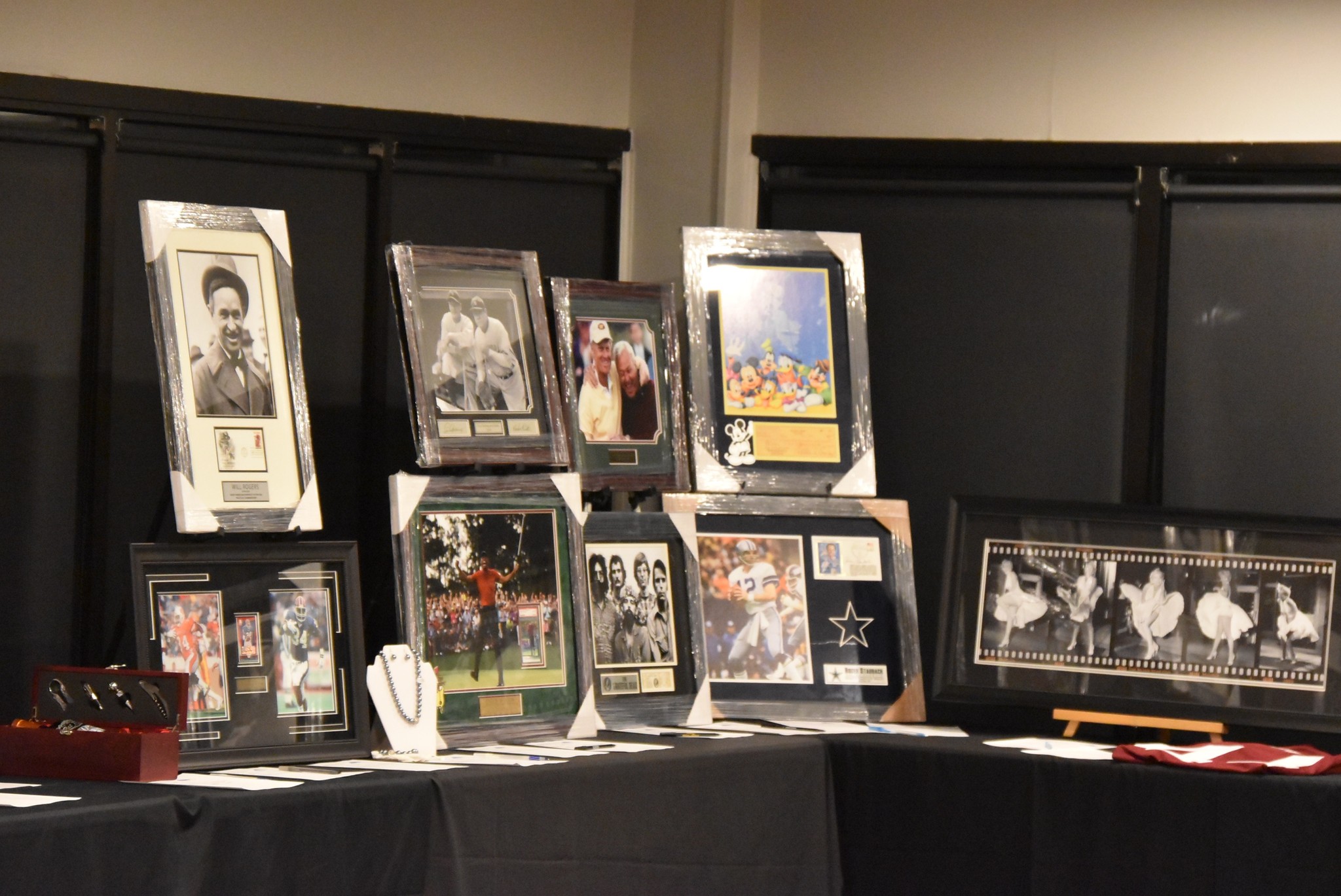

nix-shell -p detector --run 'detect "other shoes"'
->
[1066,642,1077,650]
[1145,643,1159,660]
[497,683,505,687]
[285,701,297,708]
[1227,655,1235,666]
[470,671,479,682]
[215,695,224,711]
[296,705,304,712]
[1088,645,1094,655]
[765,671,784,680]
[1206,651,1217,660]
[998,639,1010,648]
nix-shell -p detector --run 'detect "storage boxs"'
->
[0,662,189,785]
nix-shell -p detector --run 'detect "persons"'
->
[272,591,334,713]
[572,321,658,441]
[242,619,254,646]
[158,593,227,717]
[991,556,1319,666]
[697,536,809,680]
[818,543,840,574]
[587,551,674,665]
[190,258,274,416]
[425,550,559,686]
[431,290,527,411]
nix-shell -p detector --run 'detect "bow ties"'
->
[229,357,248,368]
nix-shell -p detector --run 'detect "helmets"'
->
[736,540,759,566]
[294,596,307,623]
[173,606,185,624]
[785,564,804,595]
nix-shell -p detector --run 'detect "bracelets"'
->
[379,748,419,755]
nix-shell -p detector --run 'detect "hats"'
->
[618,585,639,605]
[200,254,249,318]
[479,548,493,557]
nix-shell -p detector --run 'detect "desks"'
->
[0,724,1341,896]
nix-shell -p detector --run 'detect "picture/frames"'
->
[681,227,877,498]
[383,244,572,472]
[930,493,1341,731]
[662,492,926,724]
[139,200,324,534]
[546,275,693,495]
[389,470,598,750]
[584,508,714,727]
[127,541,372,769]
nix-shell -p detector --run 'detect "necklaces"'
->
[380,649,422,725]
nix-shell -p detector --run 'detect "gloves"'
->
[167,628,177,639]
[287,620,297,634]
